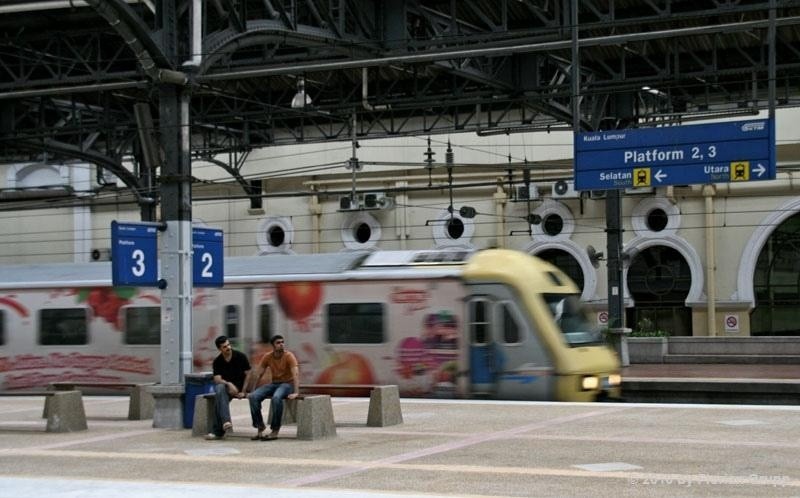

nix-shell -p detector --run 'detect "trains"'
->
[0,248,628,407]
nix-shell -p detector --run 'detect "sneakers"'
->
[205,433,216,440]
[252,435,277,441]
[224,422,232,433]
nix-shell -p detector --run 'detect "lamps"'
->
[289,74,312,108]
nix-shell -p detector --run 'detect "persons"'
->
[204,338,252,441]
[248,334,300,440]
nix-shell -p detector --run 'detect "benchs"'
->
[1,380,160,432]
[291,382,403,428]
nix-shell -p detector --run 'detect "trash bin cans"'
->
[184,371,216,429]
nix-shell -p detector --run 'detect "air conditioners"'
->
[550,180,582,200]
[515,183,537,199]
[338,190,385,209]
